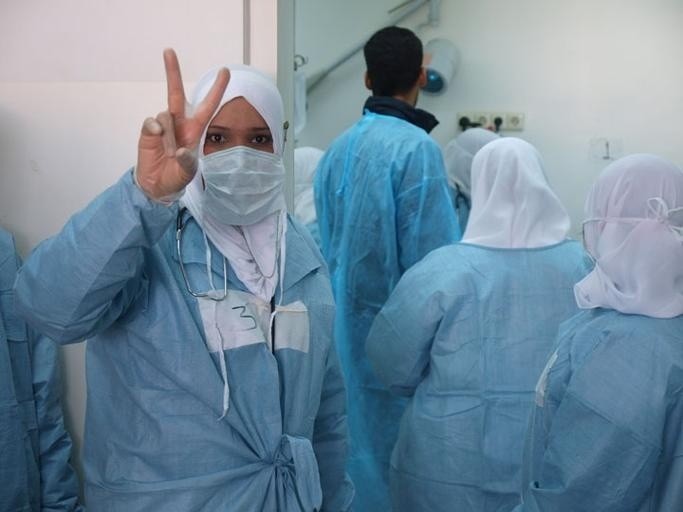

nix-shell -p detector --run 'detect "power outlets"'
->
[455,110,524,130]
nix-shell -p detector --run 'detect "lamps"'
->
[294,0,460,96]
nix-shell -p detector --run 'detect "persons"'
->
[12,48,356,510]
[438,128,505,244]
[313,25,460,508]
[366,137,600,510]
[293,146,332,266]
[1,210,78,510]
[520,155,682,511]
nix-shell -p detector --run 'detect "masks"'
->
[197,147,284,225]
